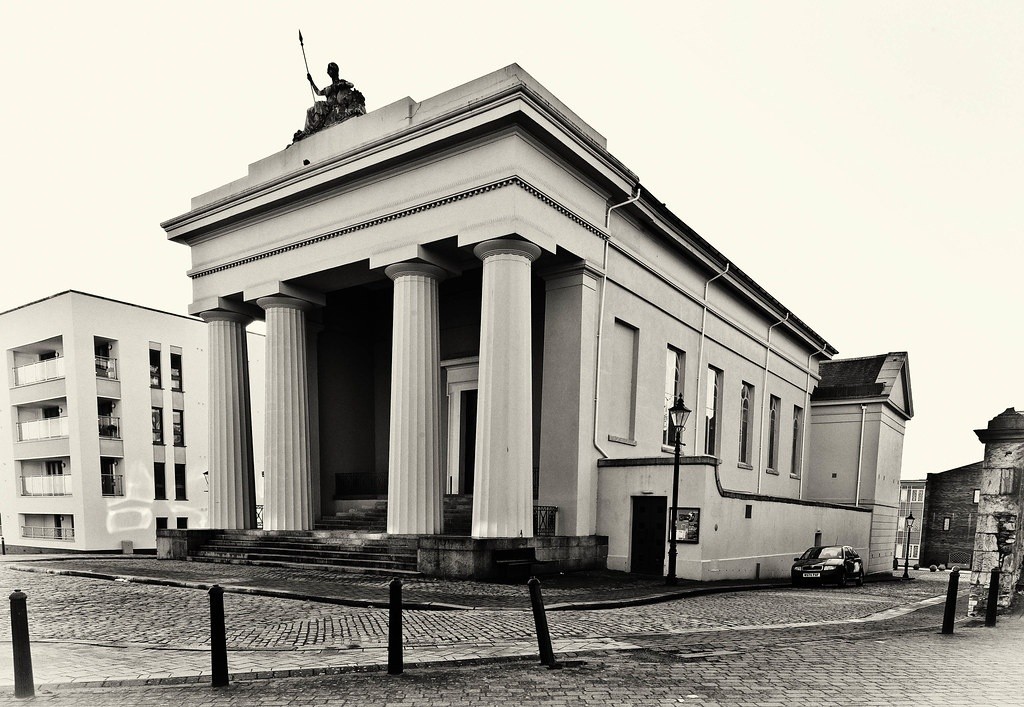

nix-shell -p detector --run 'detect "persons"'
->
[307,62,354,105]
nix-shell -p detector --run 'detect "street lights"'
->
[901,511,915,580]
[663,392,692,588]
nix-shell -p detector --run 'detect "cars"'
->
[790,544,864,589]
